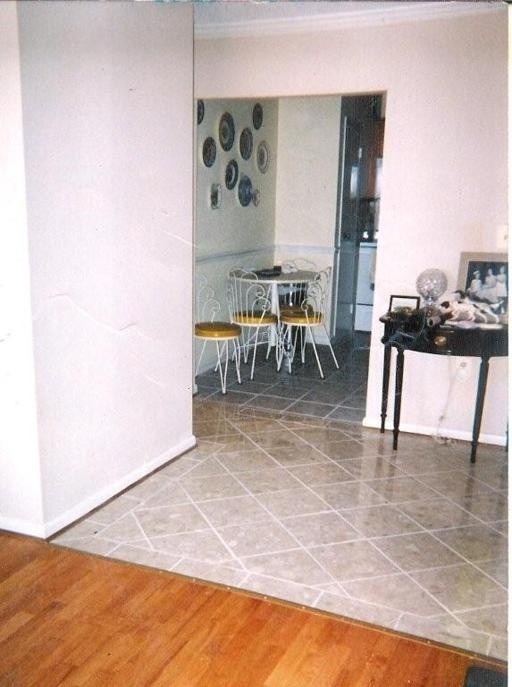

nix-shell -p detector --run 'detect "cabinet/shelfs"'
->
[352,243,376,331]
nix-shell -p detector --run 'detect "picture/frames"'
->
[456,251,508,325]
[388,295,419,317]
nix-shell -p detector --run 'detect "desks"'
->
[380,313,508,463]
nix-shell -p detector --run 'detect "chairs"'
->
[194,272,242,396]
[213,258,340,381]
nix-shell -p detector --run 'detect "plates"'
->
[197,98,271,209]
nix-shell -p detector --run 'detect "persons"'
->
[470,266,507,314]
